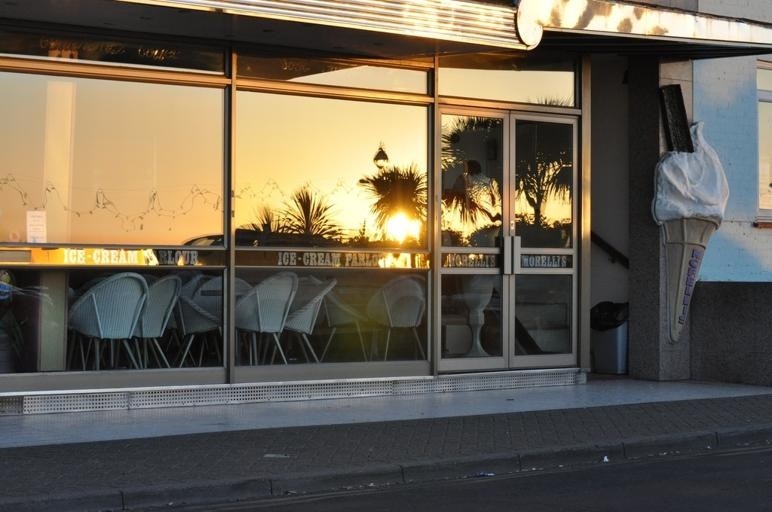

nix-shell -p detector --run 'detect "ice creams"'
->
[651,120,730,345]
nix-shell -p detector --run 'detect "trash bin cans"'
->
[591,300,628,375]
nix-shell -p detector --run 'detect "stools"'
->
[441,313,473,357]
